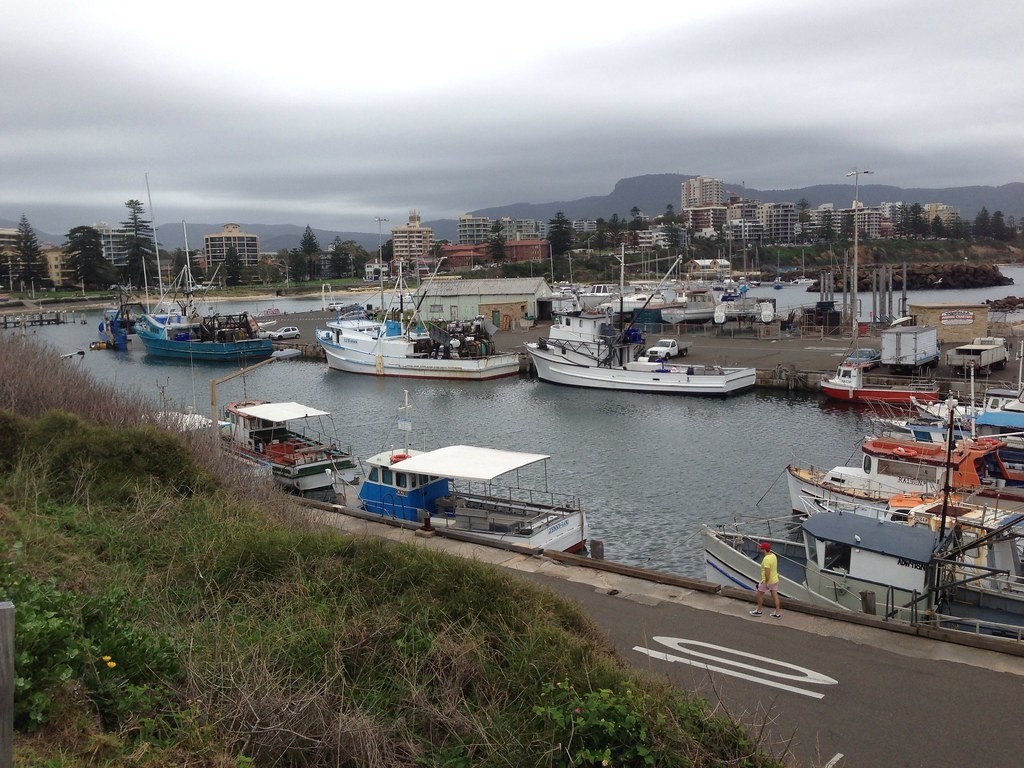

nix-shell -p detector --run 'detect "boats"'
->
[522,309,756,397]
[784,435,1024,596]
[866,375,1023,452]
[820,365,941,405]
[356,444,590,556]
[97,301,151,352]
[703,511,1024,646]
[316,316,520,383]
[141,393,358,491]
[133,311,274,360]
[551,276,816,323]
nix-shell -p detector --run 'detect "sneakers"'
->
[749,609,762,616]
[770,613,782,619]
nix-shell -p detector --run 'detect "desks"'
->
[283,451,314,465]
[485,513,526,528]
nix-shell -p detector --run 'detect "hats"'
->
[757,542,771,550]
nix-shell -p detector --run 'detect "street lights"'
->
[846,166,875,346]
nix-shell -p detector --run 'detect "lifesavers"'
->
[892,447,917,457]
[980,438,999,445]
[390,454,412,464]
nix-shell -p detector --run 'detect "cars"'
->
[846,347,881,372]
[258,326,300,341]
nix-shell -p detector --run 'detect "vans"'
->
[329,302,344,312]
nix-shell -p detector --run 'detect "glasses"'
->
[760,548,765,550]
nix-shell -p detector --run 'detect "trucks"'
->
[945,336,1011,377]
[880,325,943,374]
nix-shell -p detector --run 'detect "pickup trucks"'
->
[646,338,694,361]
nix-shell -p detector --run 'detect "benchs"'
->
[287,437,307,448]
[265,443,295,465]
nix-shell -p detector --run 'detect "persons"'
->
[749,542,782,619]
